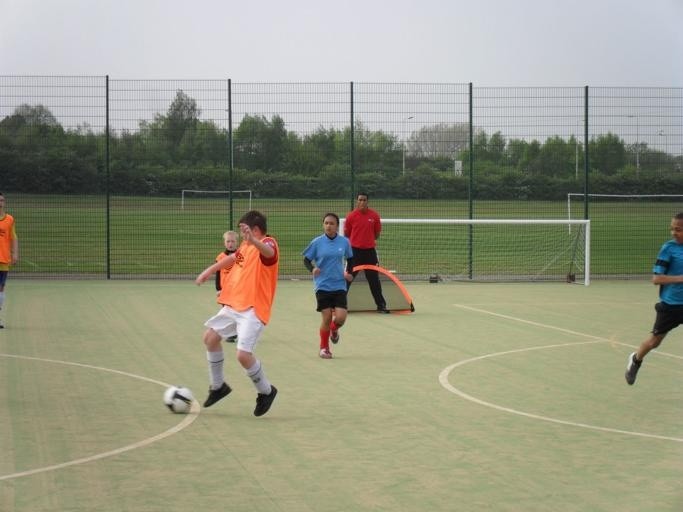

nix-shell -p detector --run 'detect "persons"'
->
[192,207,278,416]
[344,192,387,313]
[214,229,240,344]
[624,213,683,385]
[300,212,352,359]
[0,196,18,330]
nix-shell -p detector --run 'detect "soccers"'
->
[164,386,193,413]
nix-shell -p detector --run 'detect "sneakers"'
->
[253,384,277,418]
[376,306,389,313]
[319,348,332,359]
[225,334,237,343]
[625,353,642,385]
[330,321,339,344]
[203,383,232,408]
